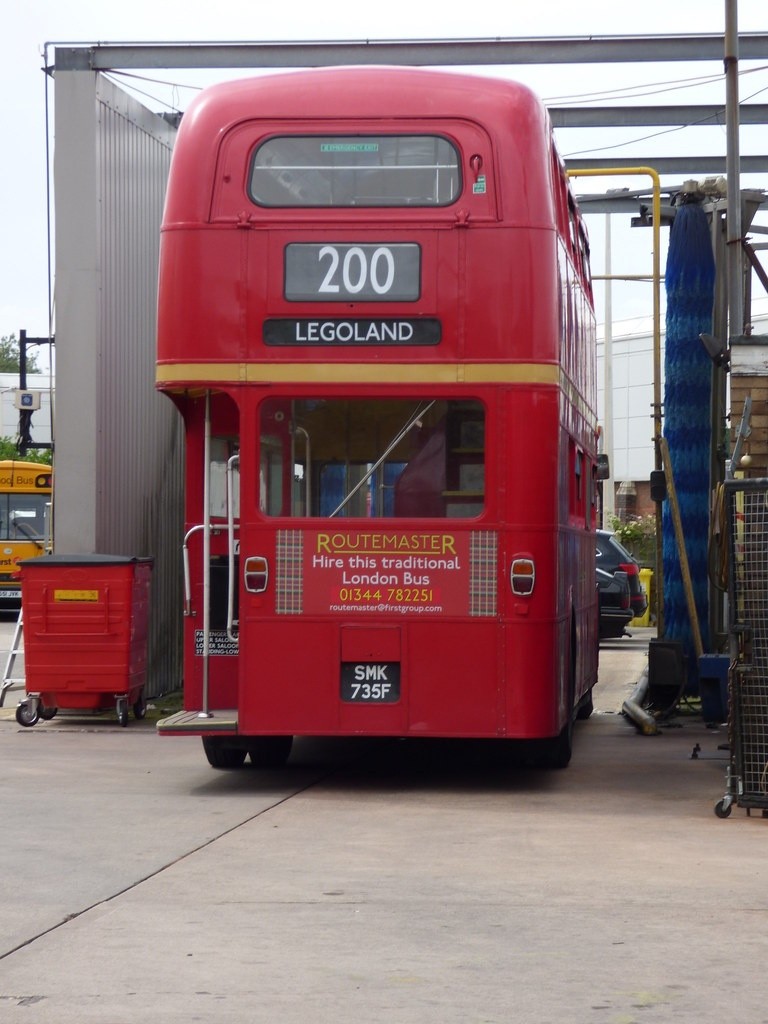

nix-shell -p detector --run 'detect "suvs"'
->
[596,528,648,635]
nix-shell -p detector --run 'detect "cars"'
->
[595,566,634,640]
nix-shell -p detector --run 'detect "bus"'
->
[150,64,612,773]
[0,458,56,616]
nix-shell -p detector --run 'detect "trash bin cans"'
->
[12,554,154,727]
[628,568,654,627]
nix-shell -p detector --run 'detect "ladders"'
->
[0,604,27,708]
